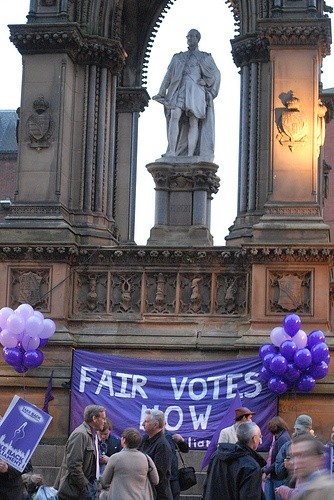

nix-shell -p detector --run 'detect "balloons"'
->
[283,314,302,338]
[260,330,331,394]
[0,304,56,373]
[270,327,291,347]
[293,330,307,349]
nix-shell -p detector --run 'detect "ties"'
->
[95,434,99,481]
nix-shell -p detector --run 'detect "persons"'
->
[0,414,42,500]
[139,410,173,500]
[262,416,290,500]
[275,415,334,500]
[97,418,123,476]
[54,405,106,500]
[164,423,189,500]
[206,422,267,500]
[152,29,215,157]
[218,407,256,444]
[102,428,159,500]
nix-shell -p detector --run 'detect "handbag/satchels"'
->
[179,466,198,490]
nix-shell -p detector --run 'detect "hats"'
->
[233,407,256,419]
